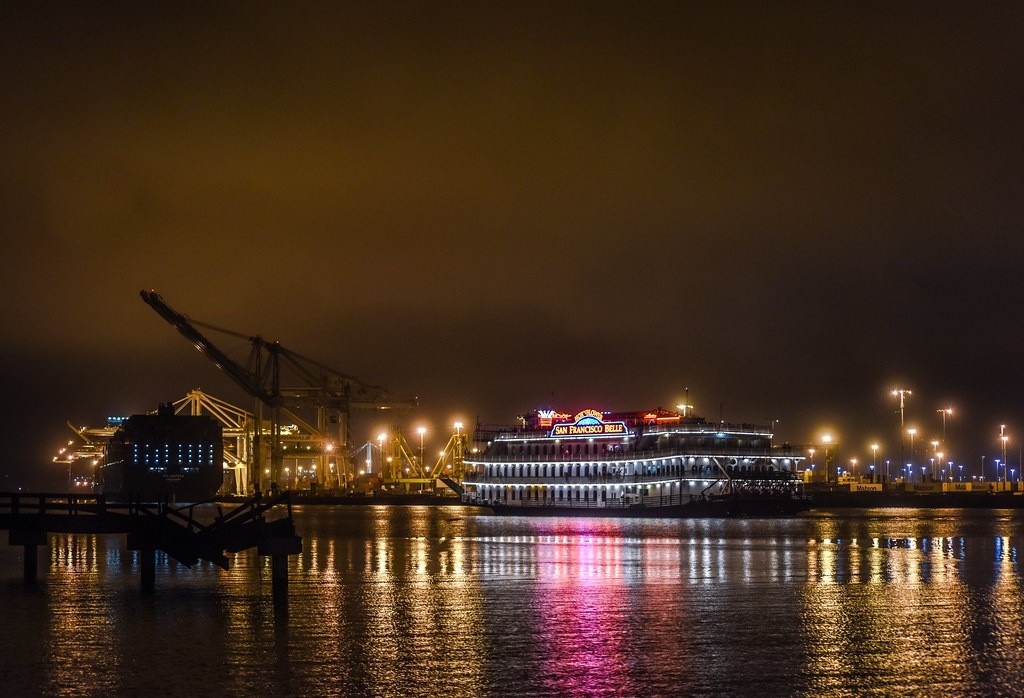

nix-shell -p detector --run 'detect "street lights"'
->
[50,385,1024,491]
[936,408,953,444]
[1000,424,1007,481]
[890,388,913,483]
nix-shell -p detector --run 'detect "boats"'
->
[461,407,813,519]
